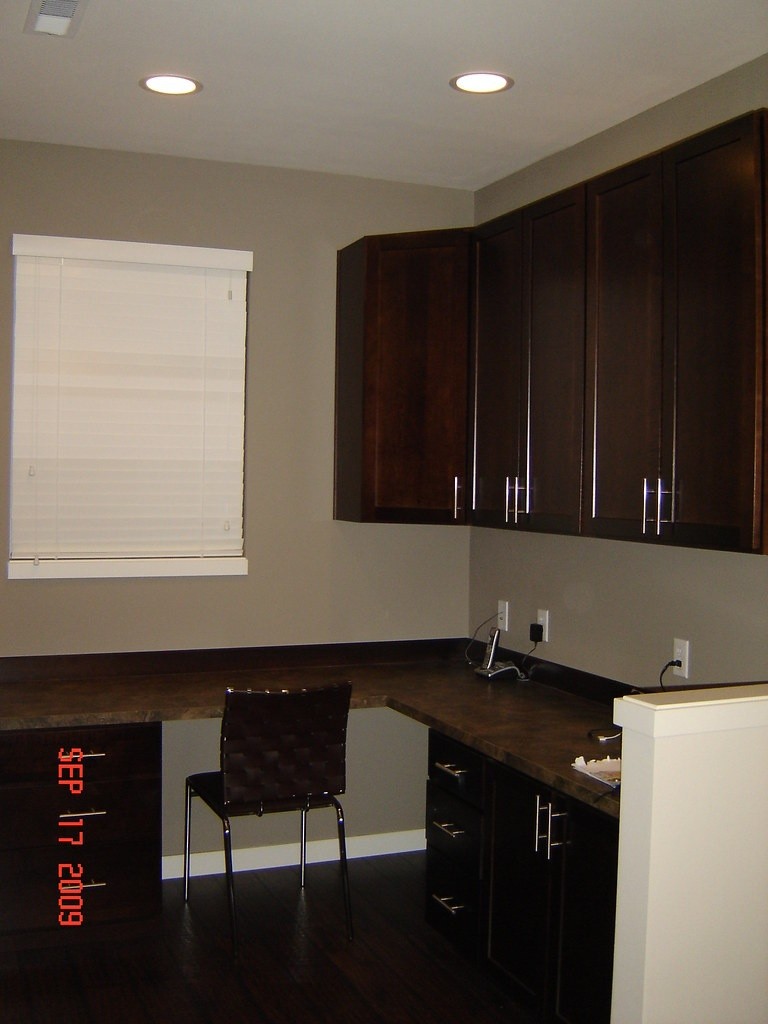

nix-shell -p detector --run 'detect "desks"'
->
[0,657,621,816]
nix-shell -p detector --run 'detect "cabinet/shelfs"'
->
[468,174,583,538]
[0,723,164,933]
[334,228,469,525]
[422,730,617,1023]
[582,108,767,555]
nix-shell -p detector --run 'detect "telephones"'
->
[473,626,526,679]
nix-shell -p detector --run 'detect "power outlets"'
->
[496,599,508,630]
[537,609,549,643]
[674,638,690,680]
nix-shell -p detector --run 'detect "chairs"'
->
[182,681,354,956]
